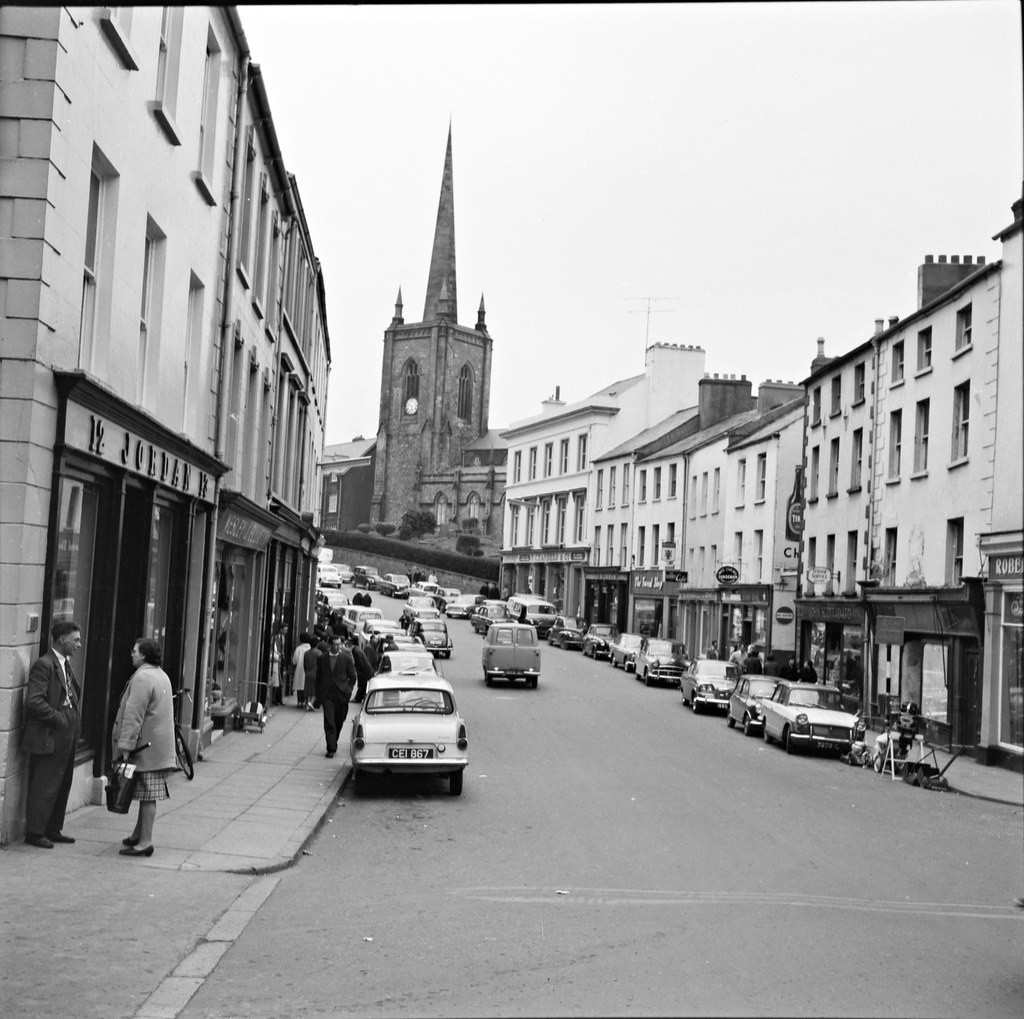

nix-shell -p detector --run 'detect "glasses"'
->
[131,650,136,653]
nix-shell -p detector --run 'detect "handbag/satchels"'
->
[104,759,137,814]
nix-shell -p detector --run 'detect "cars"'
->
[679,657,742,712]
[759,679,866,756]
[333,605,437,676]
[317,559,555,637]
[545,615,693,684]
[728,674,787,739]
[482,624,541,687]
[351,672,468,793]
[400,606,451,660]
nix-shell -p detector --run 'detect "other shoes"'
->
[350,699,362,703]
[279,701,285,706]
[326,749,337,758]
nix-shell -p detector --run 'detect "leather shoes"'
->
[24,836,54,848]
[119,837,154,857]
[49,832,75,842]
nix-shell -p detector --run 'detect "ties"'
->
[65,659,77,707]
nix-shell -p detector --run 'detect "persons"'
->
[271,591,374,710]
[480,581,509,601]
[405,568,438,584]
[706,640,818,684]
[398,609,411,631]
[105,636,177,857]
[313,635,358,757]
[19,621,84,848]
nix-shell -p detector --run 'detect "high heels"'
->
[297,701,315,712]
